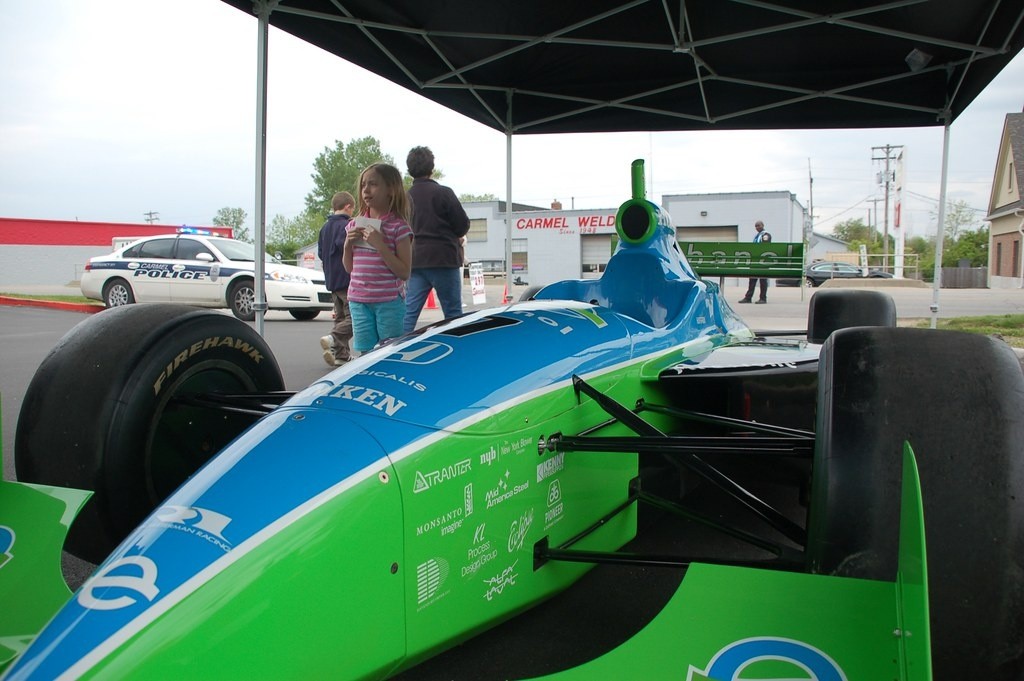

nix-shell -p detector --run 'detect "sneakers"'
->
[321,334,337,367]
[336,356,356,366]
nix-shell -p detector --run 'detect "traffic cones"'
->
[424,288,440,309]
[502,280,508,304]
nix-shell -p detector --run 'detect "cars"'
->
[79,227,336,322]
[776,260,893,288]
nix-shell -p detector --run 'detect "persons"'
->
[405,146,470,334]
[738,220,772,304]
[317,192,356,369]
[342,164,414,354]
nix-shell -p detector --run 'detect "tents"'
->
[218,0,1024,345]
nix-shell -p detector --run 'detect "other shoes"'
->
[738,298,751,303]
[755,300,766,304]
